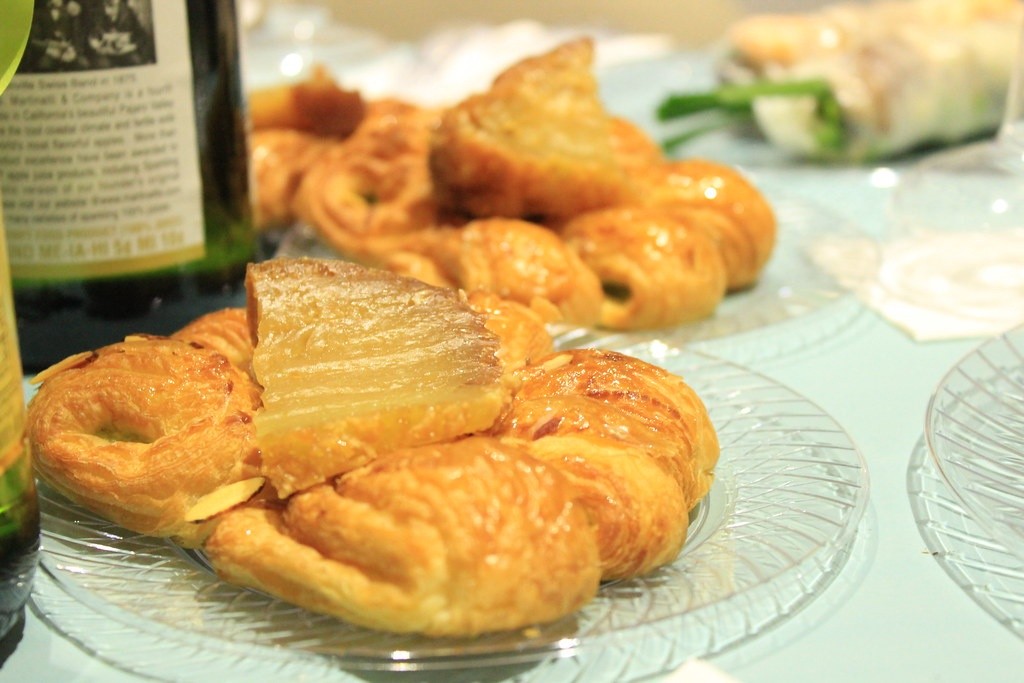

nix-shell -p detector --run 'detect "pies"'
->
[654,0,1024,166]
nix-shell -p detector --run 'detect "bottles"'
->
[0,222,42,639]
[0,0,256,376]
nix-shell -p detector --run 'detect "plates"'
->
[39,334,861,671]
[270,177,876,345]
[926,321,1024,566]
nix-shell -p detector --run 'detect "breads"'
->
[235,36,777,330]
[22,301,721,635]
